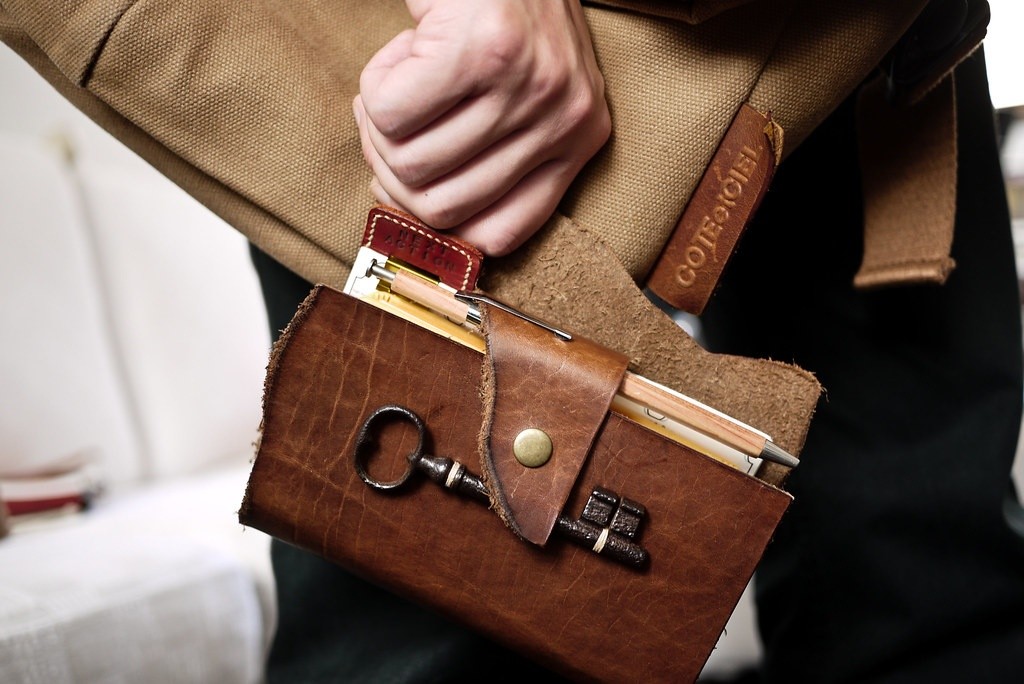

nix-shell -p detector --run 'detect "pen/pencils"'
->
[365,258,801,471]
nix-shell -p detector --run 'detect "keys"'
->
[353,402,648,567]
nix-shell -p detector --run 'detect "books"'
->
[343,247,773,477]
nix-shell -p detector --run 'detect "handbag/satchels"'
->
[236,212,824,683]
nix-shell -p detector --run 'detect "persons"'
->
[248,0,1024,684]
